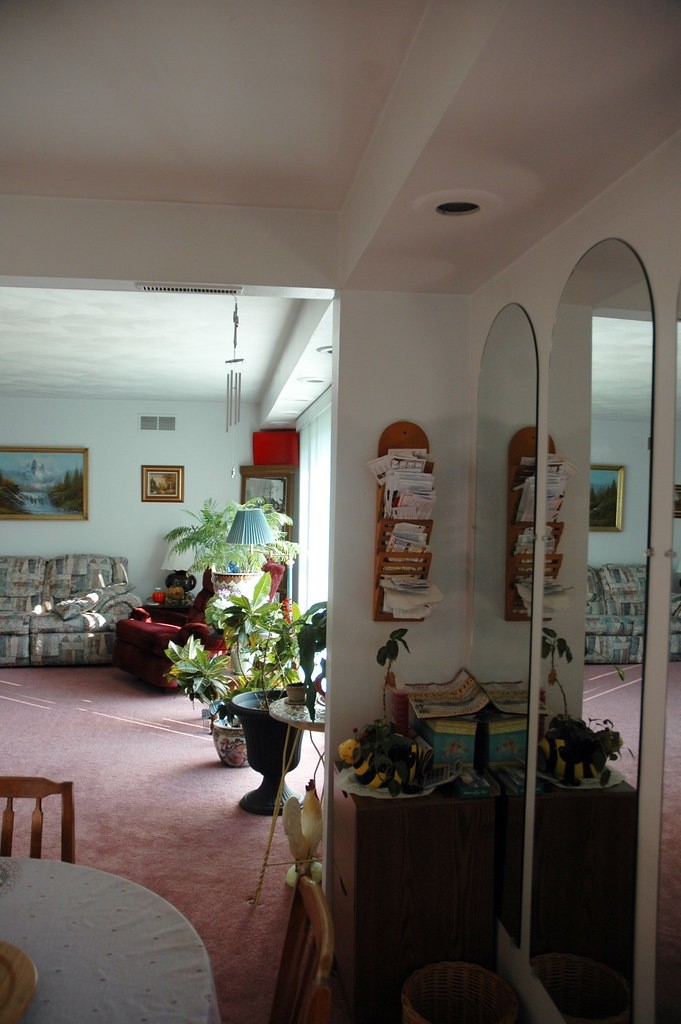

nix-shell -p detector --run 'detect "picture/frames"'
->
[589,463,624,535]
[141,465,185,503]
[0,447,90,521]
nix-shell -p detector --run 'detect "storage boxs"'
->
[251,431,299,466]
[407,716,479,771]
[480,713,526,769]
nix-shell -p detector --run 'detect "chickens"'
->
[282,778,323,877]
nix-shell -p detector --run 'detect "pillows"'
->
[88,577,136,613]
[49,587,112,620]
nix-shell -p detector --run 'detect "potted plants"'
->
[161,496,327,816]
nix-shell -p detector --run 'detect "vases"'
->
[336,735,417,789]
[542,735,601,781]
[287,683,307,702]
[229,637,256,674]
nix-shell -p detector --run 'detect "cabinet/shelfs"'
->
[330,759,500,1024]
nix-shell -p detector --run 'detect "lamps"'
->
[160,535,198,593]
[226,509,277,556]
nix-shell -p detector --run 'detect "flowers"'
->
[541,623,622,788]
[352,628,420,797]
[205,571,293,650]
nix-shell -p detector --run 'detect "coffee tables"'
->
[143,600,194,614]
[209,669,281,735]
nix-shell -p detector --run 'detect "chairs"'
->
[268,875,334,1024]
[0,776,76,863]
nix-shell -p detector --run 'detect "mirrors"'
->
[461,241,541,950]
[530,237,657,1024]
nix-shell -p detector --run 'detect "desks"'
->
[0,856,221,1024]
[254,693,326,905]
[493,771,639,1024]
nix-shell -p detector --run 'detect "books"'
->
[478,681,554,714]
[405,669,490,718]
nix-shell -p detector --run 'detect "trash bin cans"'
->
[402,962,519,1024]
[530,953,632,1024]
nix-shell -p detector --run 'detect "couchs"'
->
[115,555,286,693]
[585,564,681,662]
[0,554,142,669]
